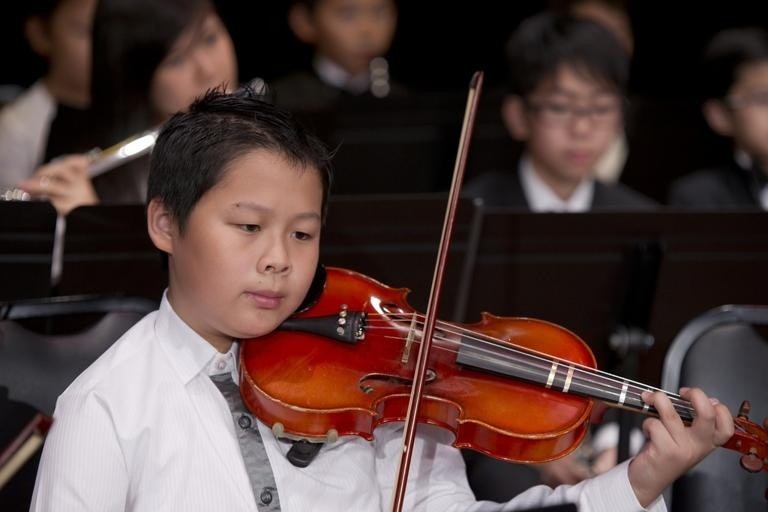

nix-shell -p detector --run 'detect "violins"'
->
[237,263,768,476]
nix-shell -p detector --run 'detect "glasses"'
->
[528,102,619,121]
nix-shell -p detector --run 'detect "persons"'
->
[14,0,239,219]
[29,78,736,512]
[458,1,655,216]
[1,1,96,196]
[272,1,438,135]
[668,29,768,219]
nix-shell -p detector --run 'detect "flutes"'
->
[0,80,267,204]
